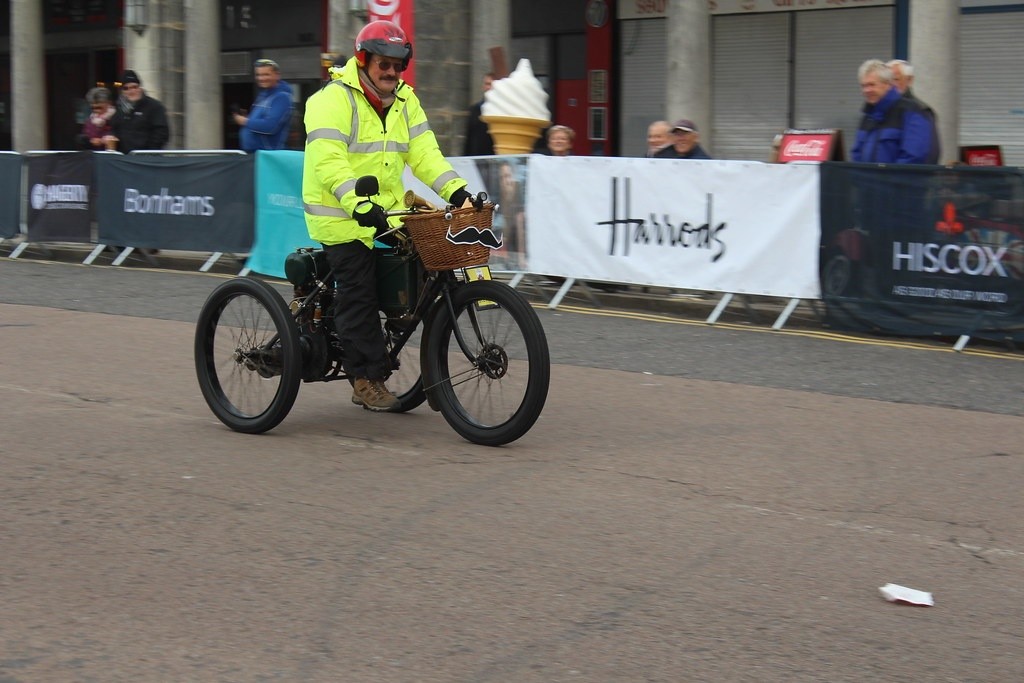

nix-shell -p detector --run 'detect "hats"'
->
[120,70,140,84]
[671,120,700,134]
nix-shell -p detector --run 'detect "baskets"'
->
[400,203,494,271]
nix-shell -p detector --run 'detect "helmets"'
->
[355,20,413,71]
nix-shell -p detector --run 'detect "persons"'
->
[643,120,674,158]
[108,68,171,254]
[848,54,943,305]
[302,20,476,412]
[546,125,576,156]
[487,161,526,271]
[68,84,127,253]
[655,119,712,159]
[232,58,296,277]
[461,70,499,157]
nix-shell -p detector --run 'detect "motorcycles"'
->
[193,172,549,446]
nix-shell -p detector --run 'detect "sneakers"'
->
[351,376,401,411]
[384,314,417,332]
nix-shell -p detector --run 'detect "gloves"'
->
[353,201,389,229]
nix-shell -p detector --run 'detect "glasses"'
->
[123,85,138,91]
[371,58,406,72]
[90,106,103,110]
